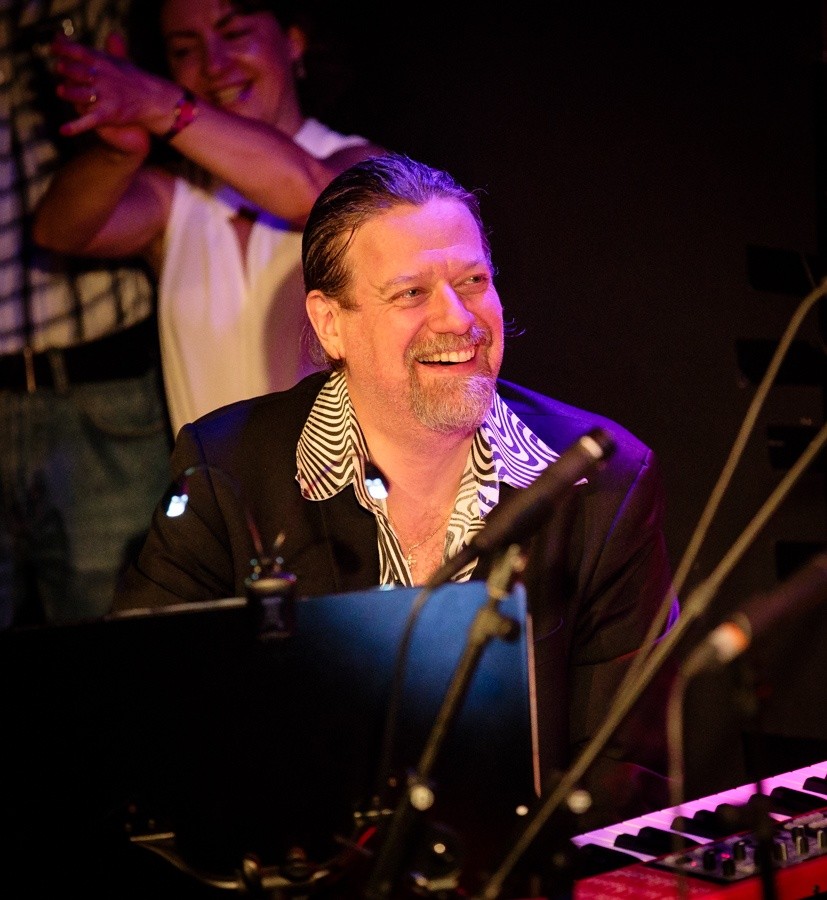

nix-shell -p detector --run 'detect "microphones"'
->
[685,554,827,675]
[426,427,615,591]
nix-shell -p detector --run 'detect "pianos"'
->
[567,760,827,900]
